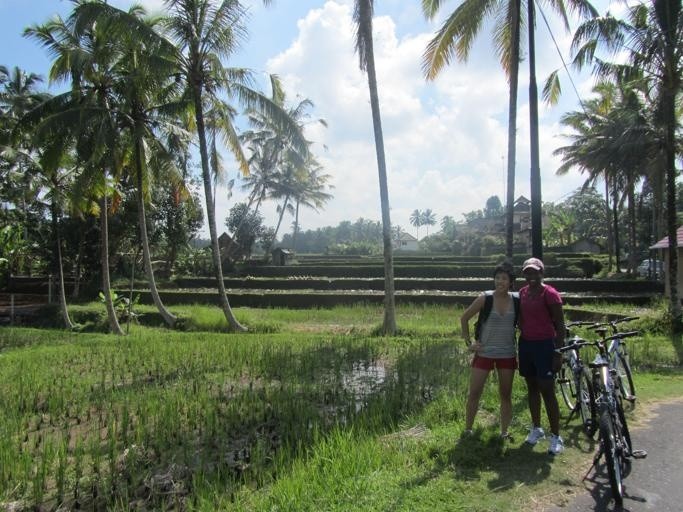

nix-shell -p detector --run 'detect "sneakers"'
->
[524,427,546,445]
[547,433,564,456]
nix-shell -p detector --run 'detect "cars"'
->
[636,259,665,278]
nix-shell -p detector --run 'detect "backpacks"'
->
[474,295,520,340]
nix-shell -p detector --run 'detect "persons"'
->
[514,257,566,456]
[460,261,521,441]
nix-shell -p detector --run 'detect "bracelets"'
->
[550,348,563,355]
[465,340,471,346]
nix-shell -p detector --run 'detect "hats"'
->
[521,257,544,272]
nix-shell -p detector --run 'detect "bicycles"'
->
[549,315,649,508]
